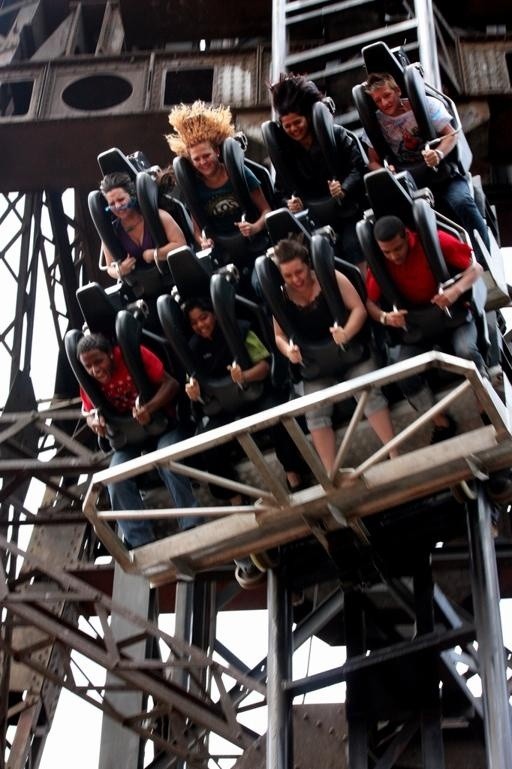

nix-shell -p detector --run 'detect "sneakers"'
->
[286,477,304,494]
[428,416,457,446]
[240,492,248,506]
[481,411,491,426]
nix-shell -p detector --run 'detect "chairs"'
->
[173,137,274,251]
[253,208,386,428]
[354,41,473,177]
[157,246,290,458]
[64,283,184,486]
[356,168,489,397]
[258,104,370,222]
[88,147,201,283]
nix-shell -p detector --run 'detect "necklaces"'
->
[119,218,142,232]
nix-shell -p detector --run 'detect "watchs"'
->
[378,311,389,325]
[434,148,447,165]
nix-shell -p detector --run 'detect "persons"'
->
[77,336,204,548]
[99,172,187,336]
[272,241,398,479]
[185,303,312,505]
[267,74,365,236]
[165,100,272,292]
[367,217,491,445]
[363,74,491,272]
